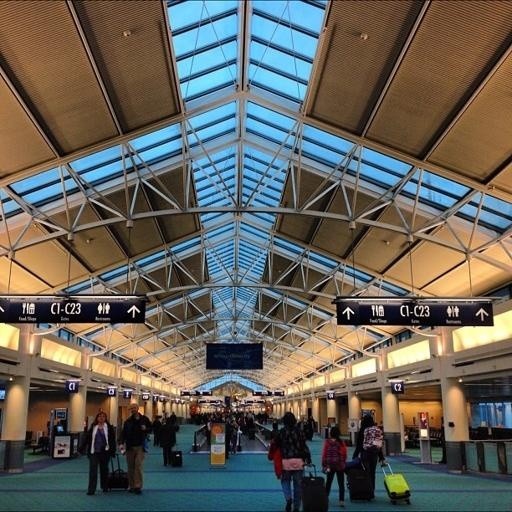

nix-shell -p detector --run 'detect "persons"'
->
[271,412,312,512]
[321,427,347,506]
[352,414,386,499]
[152,411,179,467]
[190,406,317,456]
[119,404,152,494]
[76,412,116,495]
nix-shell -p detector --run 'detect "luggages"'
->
[105,453,129,491]
[381,462,412,505]
[298,463,329,511]
[169,445,183,468]
[347,475,375,502]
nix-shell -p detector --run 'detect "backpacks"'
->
[362,424,385,454]
[324,438,344,465]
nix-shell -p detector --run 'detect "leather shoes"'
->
[128,487,134,493]
[86,490,95,495]
[135,487,142,495]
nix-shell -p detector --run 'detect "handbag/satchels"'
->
[345,457,369,477]
[282,458,304,472]
[143,436,152,453]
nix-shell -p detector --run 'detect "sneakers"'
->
[285,498,293,512]
[338,501,345,507]
